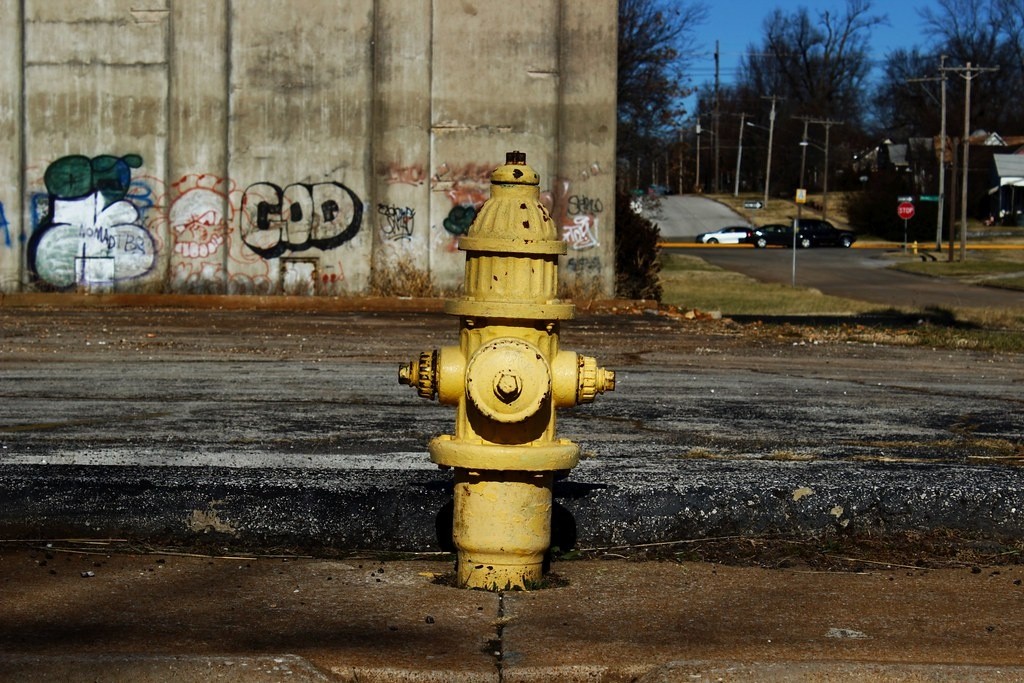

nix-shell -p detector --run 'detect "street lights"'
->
[747,122,774,208]
[799,140,828,223]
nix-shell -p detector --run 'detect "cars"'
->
[696,225,769,250]
[755,223,792,248]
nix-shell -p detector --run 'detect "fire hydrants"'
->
[396,149,616,593]
[911,240,918,255]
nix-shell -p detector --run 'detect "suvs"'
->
[790,219,858,250]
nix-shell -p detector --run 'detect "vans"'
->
[744,200,763,210]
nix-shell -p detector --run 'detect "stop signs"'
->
[897,202,915,221]
